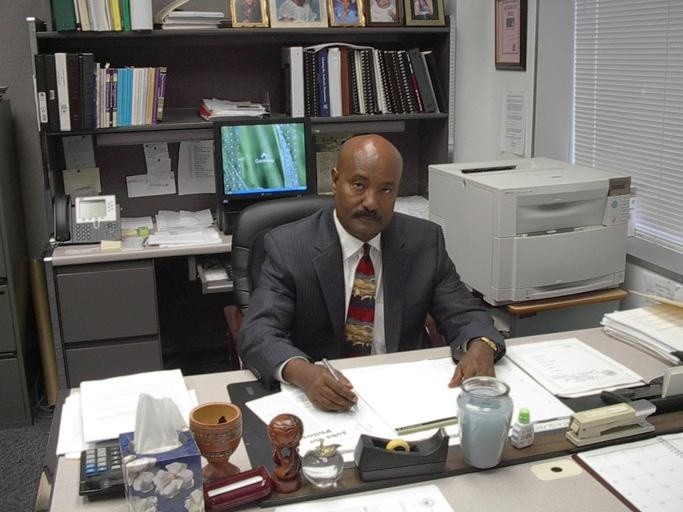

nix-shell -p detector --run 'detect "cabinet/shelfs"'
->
[23,12,453,237]
[0,82,34,435]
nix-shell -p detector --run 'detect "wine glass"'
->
[188,401,243,481]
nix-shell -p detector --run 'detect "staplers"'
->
[565,399,656,447]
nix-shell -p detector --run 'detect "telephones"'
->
[53,194,123,246]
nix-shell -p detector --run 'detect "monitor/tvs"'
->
[213,117,318,208]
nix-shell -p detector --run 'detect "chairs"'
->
[221,192,450,369]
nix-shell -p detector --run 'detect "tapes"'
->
[386,439,410,452]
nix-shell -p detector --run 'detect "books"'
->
[571,430,682,512]
[48,1,225,34]
[32,51,166,130]
[599,301,683,366]
[281,41,442,117]
[198,95,267,122]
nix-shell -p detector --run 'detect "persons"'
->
[412,0,433,21]
[237,1,261,23]
[278,0,318,23]
[369,1,398,23]
[334,0,359,25]
[234,134,509,413]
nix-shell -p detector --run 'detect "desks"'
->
[238,423,680,512]
[478,287,629,340]
[32,324,680,512]
[41,196,429,397]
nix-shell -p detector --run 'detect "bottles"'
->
[510,408,535,449]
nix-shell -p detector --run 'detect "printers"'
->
[428,157,632,307]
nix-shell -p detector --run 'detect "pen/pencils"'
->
[461,165,516,173]
[322,357,359,412]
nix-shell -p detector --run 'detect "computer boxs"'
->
[216,198,292,235]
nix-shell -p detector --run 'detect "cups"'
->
[456,376,513,469]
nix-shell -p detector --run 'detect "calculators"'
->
[79,446,124,495]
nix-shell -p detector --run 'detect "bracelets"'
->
[470,335,499,353]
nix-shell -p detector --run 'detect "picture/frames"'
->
[405,0,445,27]
[365,0,406,27]
[493,0,529,73]
[264,0,333,33]
[324,0,365,28]
[227,0,270,28]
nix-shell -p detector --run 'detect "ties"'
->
[341,243,380,358]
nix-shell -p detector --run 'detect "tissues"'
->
[117,391,207,512]
